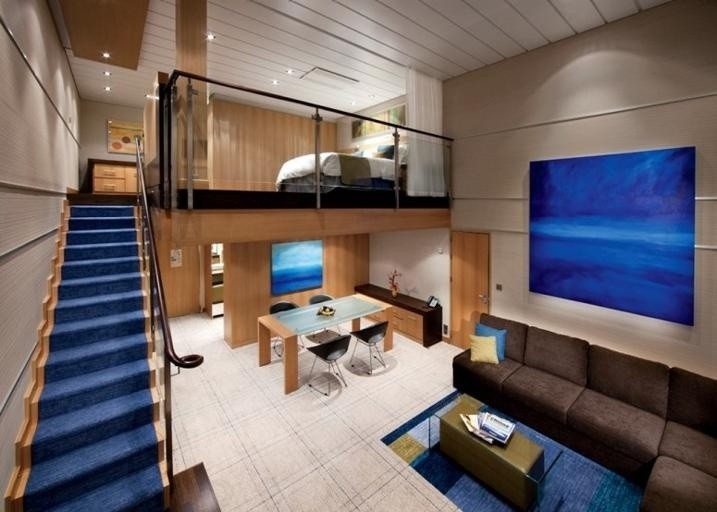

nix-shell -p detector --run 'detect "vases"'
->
[392,288,397,297]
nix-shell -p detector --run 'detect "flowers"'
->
[386,269,402,293]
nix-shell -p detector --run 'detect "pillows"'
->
[474,322,508,361]
[470,334,499,364]
[370,144,395,160]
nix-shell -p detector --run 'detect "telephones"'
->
[427,295,438,308]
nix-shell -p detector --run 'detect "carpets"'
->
[379,389,650,512]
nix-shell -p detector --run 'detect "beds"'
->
[276,134,408,193]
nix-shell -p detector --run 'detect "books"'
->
[459,412,516,445]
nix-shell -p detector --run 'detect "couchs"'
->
[452,313,716,511]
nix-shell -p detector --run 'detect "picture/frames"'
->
[350,103,407,139]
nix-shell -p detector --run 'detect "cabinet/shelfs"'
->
[354,283,442,348]
[87,158,144,194]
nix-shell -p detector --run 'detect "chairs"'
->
[306,333,351,395]
[351,321,389,375]
[307,294,341,344]
[269,301,304,357]
